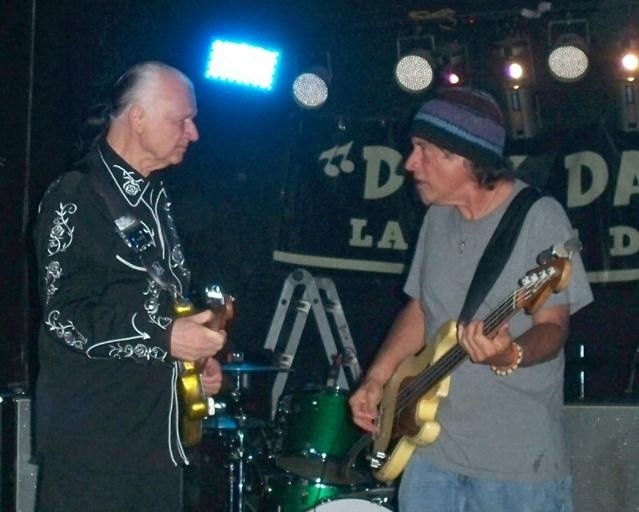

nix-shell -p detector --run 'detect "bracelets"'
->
[489,341,524,378]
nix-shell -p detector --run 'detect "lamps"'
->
[390,17,480,102]
[546,17,594,84]
[290,18,351,110]
[491,17,557,157]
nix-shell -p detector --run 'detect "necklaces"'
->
[457,178,508,255]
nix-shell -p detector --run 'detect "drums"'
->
[279,472,349,512]
[305,494,394,512]
[270,385,370,487]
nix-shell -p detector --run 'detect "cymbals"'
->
[219,362,294,374]
[201,416,267,430]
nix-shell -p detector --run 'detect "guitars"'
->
[174,283,232,445]
[370,239,582,483]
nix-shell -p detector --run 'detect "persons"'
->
[28,61,237,509]
[347,84,596,511]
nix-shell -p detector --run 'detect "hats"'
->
[411,86,507,169]
